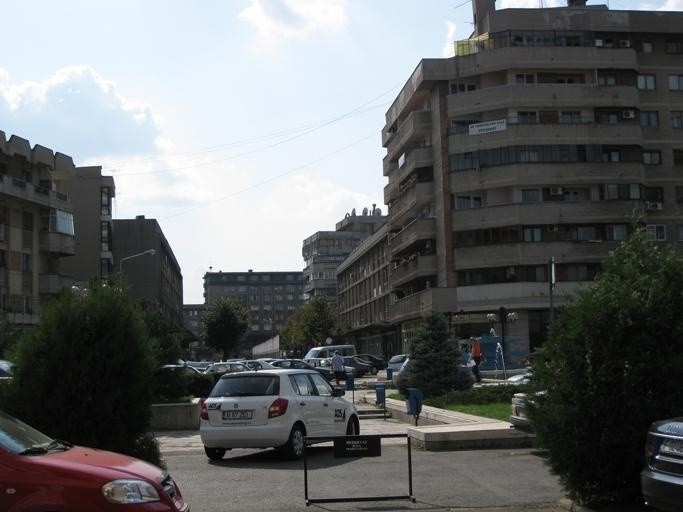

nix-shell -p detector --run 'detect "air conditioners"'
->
[618,40,631,49]
[545,224,559,232]
[650,201,662,212]
[549,185,563,196]
[622,109,635,119]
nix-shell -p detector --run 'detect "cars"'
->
[156,353,408,388]
[197,367,360,461]
[640,417,683,512]
[510,388,548,435]
[0,408,191,512]
[0,359,19,385]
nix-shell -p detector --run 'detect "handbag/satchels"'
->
[467,359,477,368]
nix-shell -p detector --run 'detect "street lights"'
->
[487,306,519,379]
[119,249,156,287]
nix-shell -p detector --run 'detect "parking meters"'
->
[374,383,385,420]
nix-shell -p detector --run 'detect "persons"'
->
[331,350,344,385]
[467,337,481,383]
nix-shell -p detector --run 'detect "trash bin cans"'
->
[375,383,386,421]
[386,367,394,389]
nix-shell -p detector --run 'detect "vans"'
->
[303,344,356,365]
